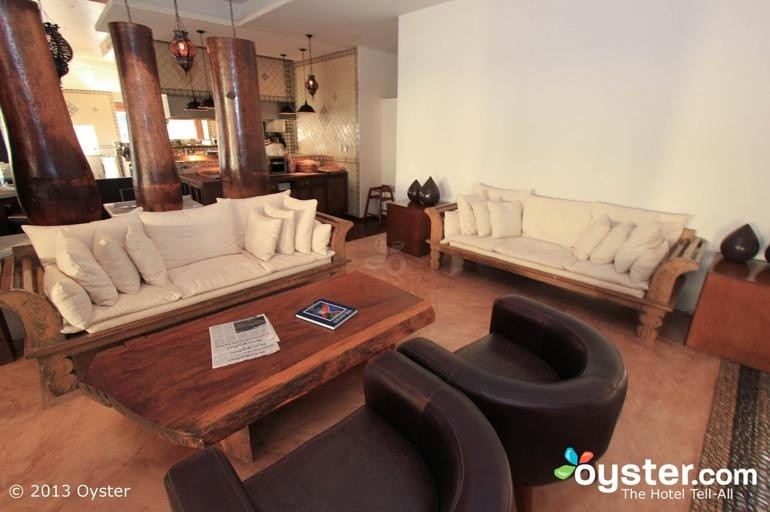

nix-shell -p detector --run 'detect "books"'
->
[297,298,357,331]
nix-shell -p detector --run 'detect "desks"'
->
[80,272,436,464]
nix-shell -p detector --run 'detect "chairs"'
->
[164,352,514,511]
[398,293,628,487]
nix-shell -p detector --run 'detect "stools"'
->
[365,185,394,226]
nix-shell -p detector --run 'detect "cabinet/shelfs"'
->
[386,198,445,257]
[685,252,770,375]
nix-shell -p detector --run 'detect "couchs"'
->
[0,190,355,397]
[424,184,709,341]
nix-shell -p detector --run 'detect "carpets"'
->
[691,354,770,512]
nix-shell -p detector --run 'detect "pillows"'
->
[444,184,593,240]
[577,214,688,283]
[21,200,241,331]
[216,189,331,261]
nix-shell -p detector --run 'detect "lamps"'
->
[37,0,73,78]
[278,34,318,115]
[170,0,217,113]
